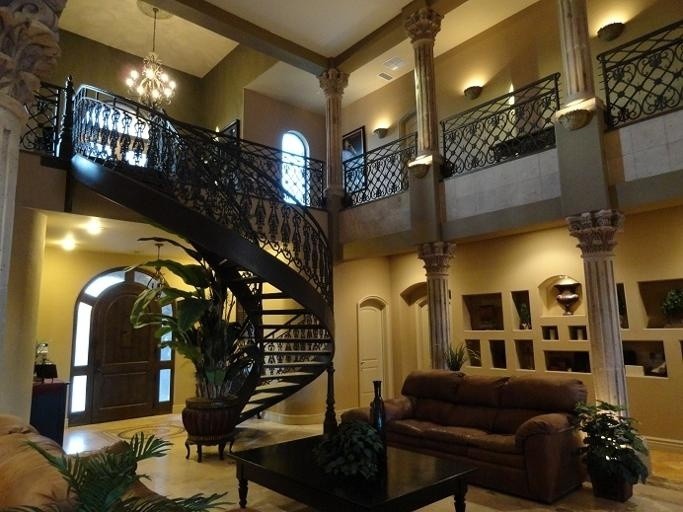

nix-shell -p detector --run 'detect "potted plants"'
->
[568,399,650,503]
[124,235,266,463]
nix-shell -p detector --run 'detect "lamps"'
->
[124,1,178,109]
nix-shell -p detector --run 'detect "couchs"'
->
[340,370,588,504]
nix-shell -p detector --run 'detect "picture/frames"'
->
[343,124,368,195]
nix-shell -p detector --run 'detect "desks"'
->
[29,378,72,448]
